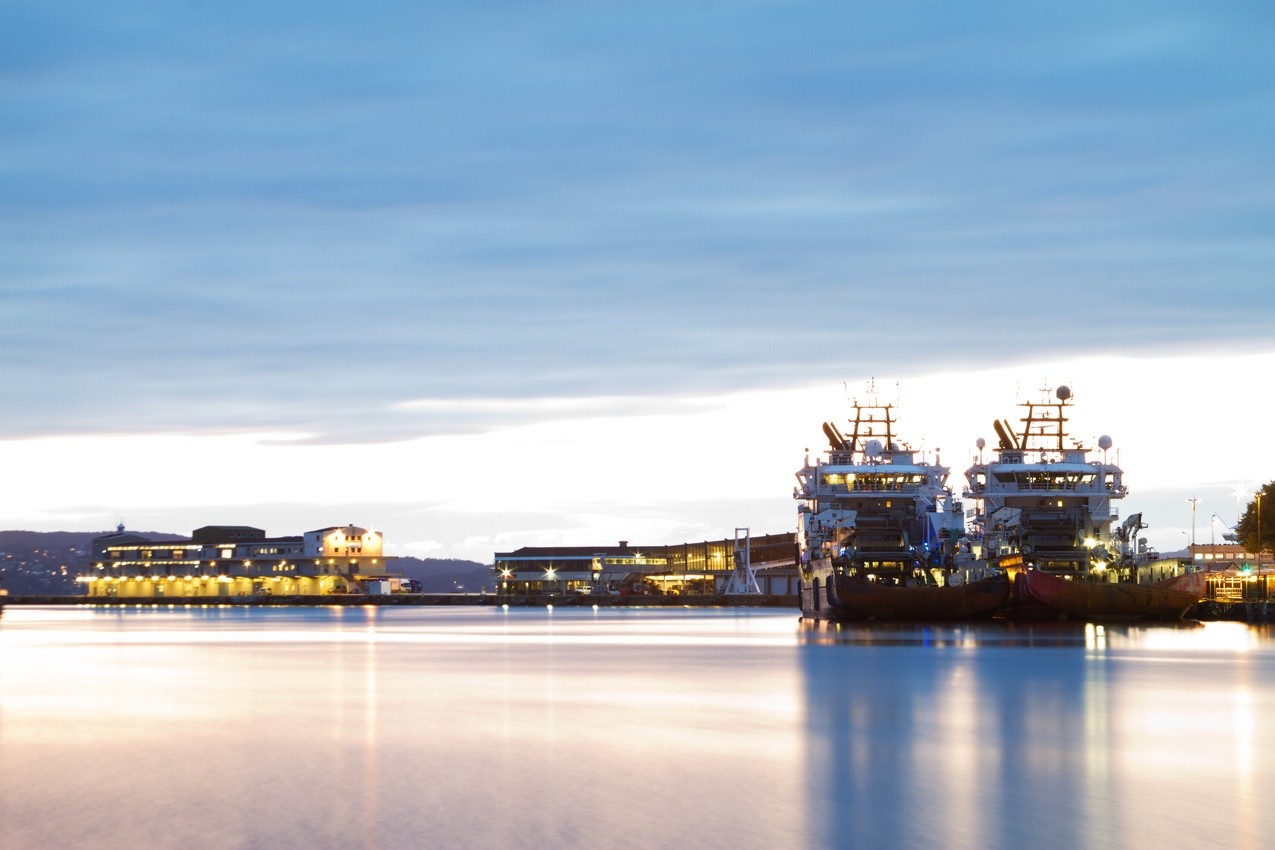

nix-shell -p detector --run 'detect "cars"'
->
[573,585,593,597]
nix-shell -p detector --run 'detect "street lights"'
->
[1187,497,1198,574]
[1250,491,1267,603]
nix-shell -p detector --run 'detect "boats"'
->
[959,381,1208,627]
[791,370,1013,623]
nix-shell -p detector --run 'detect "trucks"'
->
[387,577,423,593]
